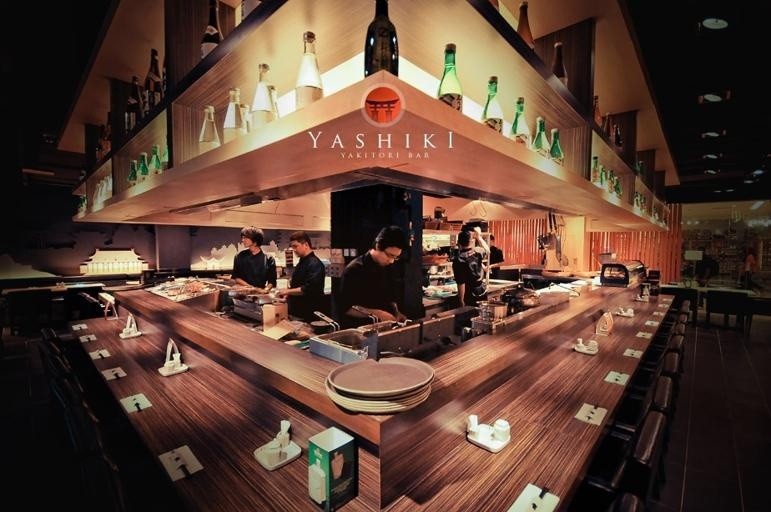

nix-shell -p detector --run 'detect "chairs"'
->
[561,291,698,511]
[36,326,187,510]
[485,257,568,293]
[645,282,771,339]
[4,268,187,407]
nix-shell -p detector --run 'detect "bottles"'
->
[123,325,138,335]
[264,430,291,464]
[365,1,399,79]
[574,338,599,351]
[197,61,280,155]
[202,0,224,58]
[298,31,322,106]
[437,1,569,166]
[495,419,511,439]
[165,352,182,372]
[595,94,674,221]
[75,45,168,213]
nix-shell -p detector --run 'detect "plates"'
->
[254,440,303,473]
[467,422,511,453]
[324,356,435,416]
[157,366,190,378]
[575,347,598,355]
[119,332,142,340]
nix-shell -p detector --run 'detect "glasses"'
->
[384,248,400,261]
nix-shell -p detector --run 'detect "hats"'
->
[459,232,470,242]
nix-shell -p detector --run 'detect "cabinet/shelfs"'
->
[55,1,686,238]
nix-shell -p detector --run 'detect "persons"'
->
[689,247,719,286]
[484,234,504,278]
[231,226,277,293]
[338,226,408,328]
[745,252,757,280]
[453,229,491,305]
[276,232,325,322]
